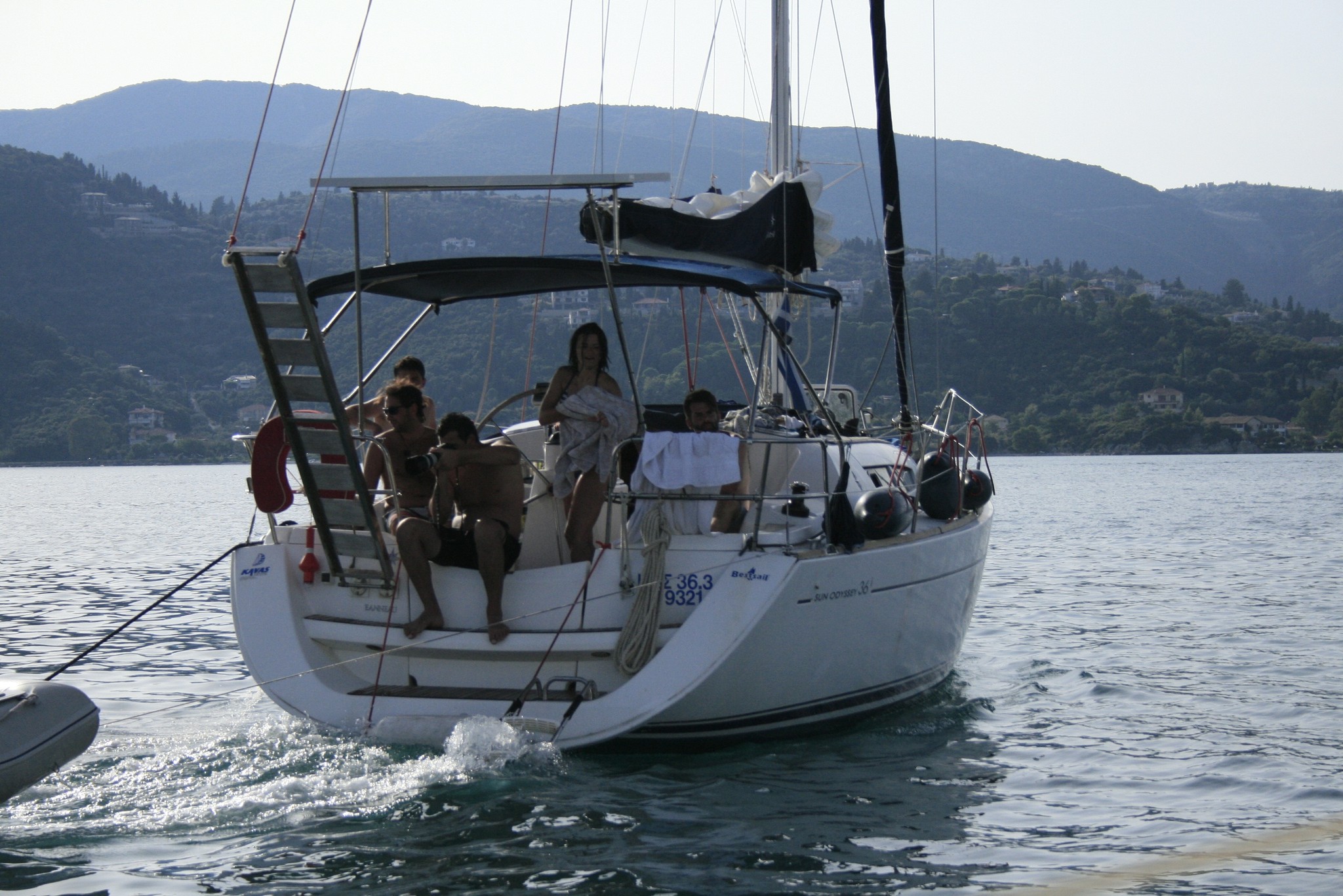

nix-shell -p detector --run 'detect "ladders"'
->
[227,246,395,590]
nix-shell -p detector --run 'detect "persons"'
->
[363,381,440,535]
[395,412,523,643]
[345,355,438,437]
[684,389,750,532]
[539,322,623,563]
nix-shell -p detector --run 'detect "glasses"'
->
[382,405,406,416]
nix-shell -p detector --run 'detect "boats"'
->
[0,678,102,803]
[229,0,999,763]
